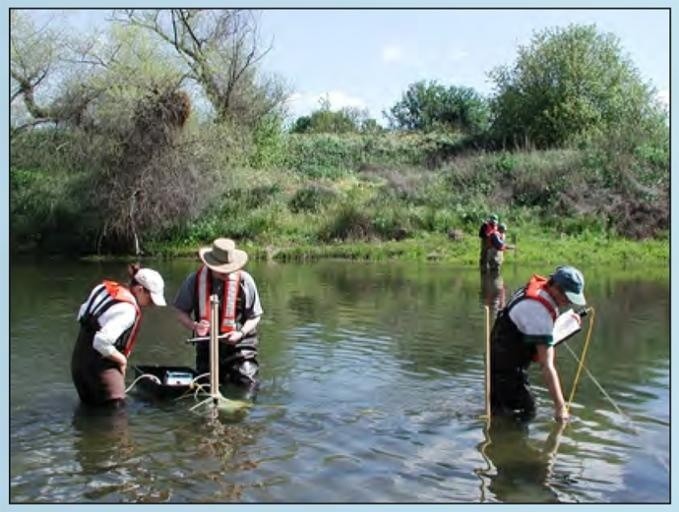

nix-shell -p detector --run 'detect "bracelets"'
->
[238,328,246,338]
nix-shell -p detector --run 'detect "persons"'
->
[483,264,587,426]
[171,236,266,392]
[487,223,516,271]
[479,268,506,320]
[70,260,168,409]
[478,215,499,270]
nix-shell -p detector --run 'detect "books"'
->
[186,333,231,343]
[551,307,580,347]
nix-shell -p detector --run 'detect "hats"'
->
[198,237,248,275]
[489,213,498,223]
[497,223,507,233]
[550,265,588,307]
[134,267,167,307]
